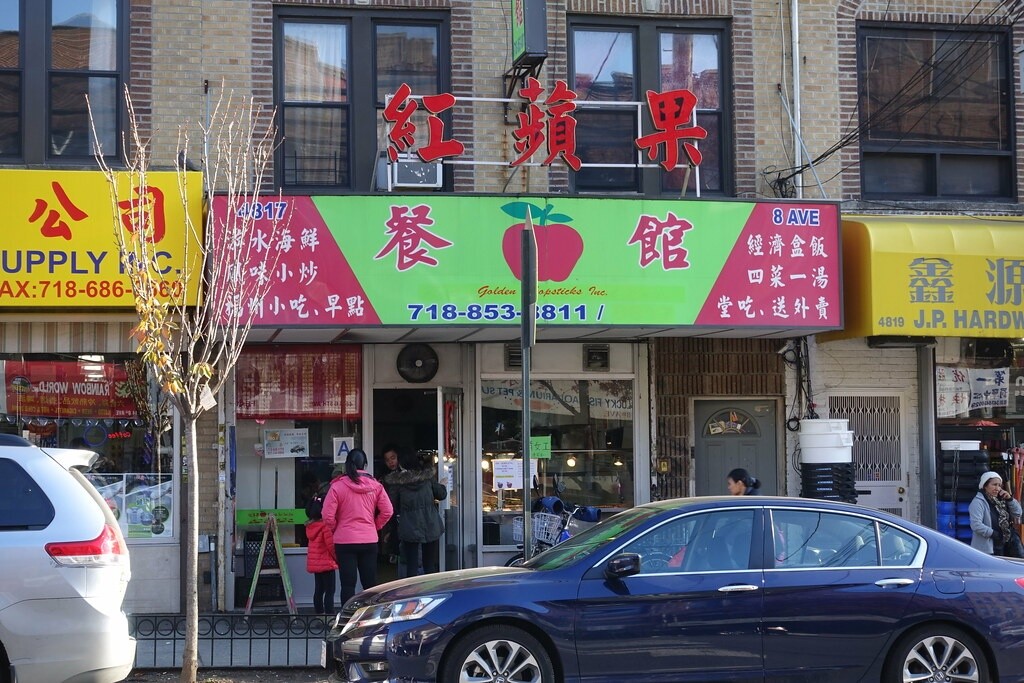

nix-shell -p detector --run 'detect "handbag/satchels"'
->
[1004,532,1024,559]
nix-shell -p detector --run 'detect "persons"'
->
[716,468,762,570]
[68,436,116,473]
[375,446,448,582]
[320,448,394,608]
[304,497,340,614]
[968,471,1022,558]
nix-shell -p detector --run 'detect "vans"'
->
[513,449,622,499]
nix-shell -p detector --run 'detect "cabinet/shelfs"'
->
[936,426,1014,545]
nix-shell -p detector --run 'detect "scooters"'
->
[502,475,678,573]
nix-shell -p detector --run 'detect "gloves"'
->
[990,530,1003,541]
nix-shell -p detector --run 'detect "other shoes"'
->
[389,554,397,564]
[315,612,335,627]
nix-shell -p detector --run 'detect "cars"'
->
[1,434,136,683]
[322,494,1024,683]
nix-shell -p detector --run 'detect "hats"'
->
[979,472,1002,489]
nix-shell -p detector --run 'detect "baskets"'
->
[534,512,564,544]
[513,516,537,543]
